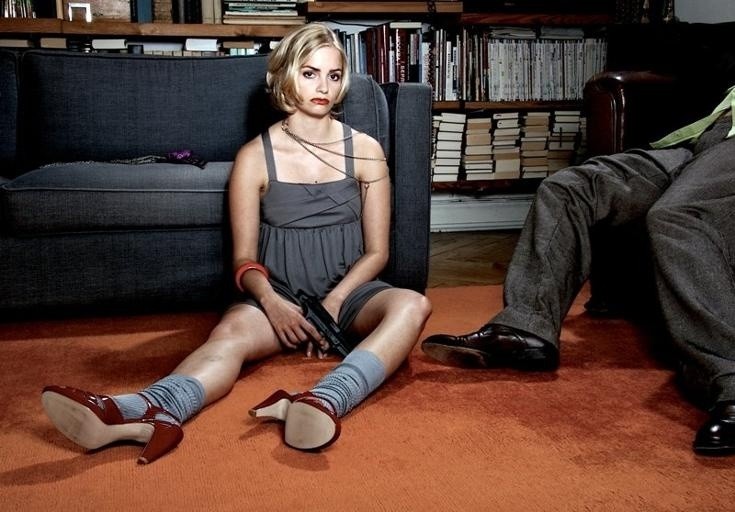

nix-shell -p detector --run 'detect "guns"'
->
[300,295,349,357]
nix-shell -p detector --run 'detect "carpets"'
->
[2,282,734,510]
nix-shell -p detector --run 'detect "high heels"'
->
[41,382,184,465]
[247,387,341,453]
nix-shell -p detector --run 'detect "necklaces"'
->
[279,117,390,219]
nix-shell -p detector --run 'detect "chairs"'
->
[578,64,734,319]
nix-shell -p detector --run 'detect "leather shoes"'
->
[692,400,735,458]
[420,323,561,373]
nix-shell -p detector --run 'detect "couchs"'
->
[2,42,437,321]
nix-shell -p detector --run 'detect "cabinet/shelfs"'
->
[2,0,308,58]
[308,0,627,194]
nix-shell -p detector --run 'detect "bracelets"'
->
[233,262,270,294]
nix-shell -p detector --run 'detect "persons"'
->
[421,77,735,457]
[40,24,434,463]
